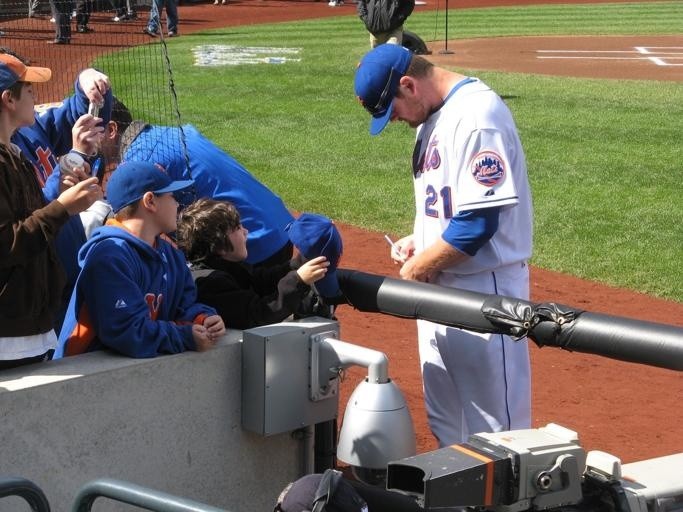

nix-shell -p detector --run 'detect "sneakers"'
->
[43,13,178,47]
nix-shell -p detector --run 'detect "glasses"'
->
[361,67,406,119]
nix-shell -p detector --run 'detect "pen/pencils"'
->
[384,235,405,263]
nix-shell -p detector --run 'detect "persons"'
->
[0,48,329,371]
[356,44,531,443]
[356,1,416,48]
[275,473,368,512]
[27,0,180,45]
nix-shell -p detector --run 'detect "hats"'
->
[283,209,347,274]
[102,159,198,218]
[0,53,51,90]
[350,41,413,136]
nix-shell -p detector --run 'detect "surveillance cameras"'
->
[332,380,413,489]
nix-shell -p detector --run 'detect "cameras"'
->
[87,97,105,119]
